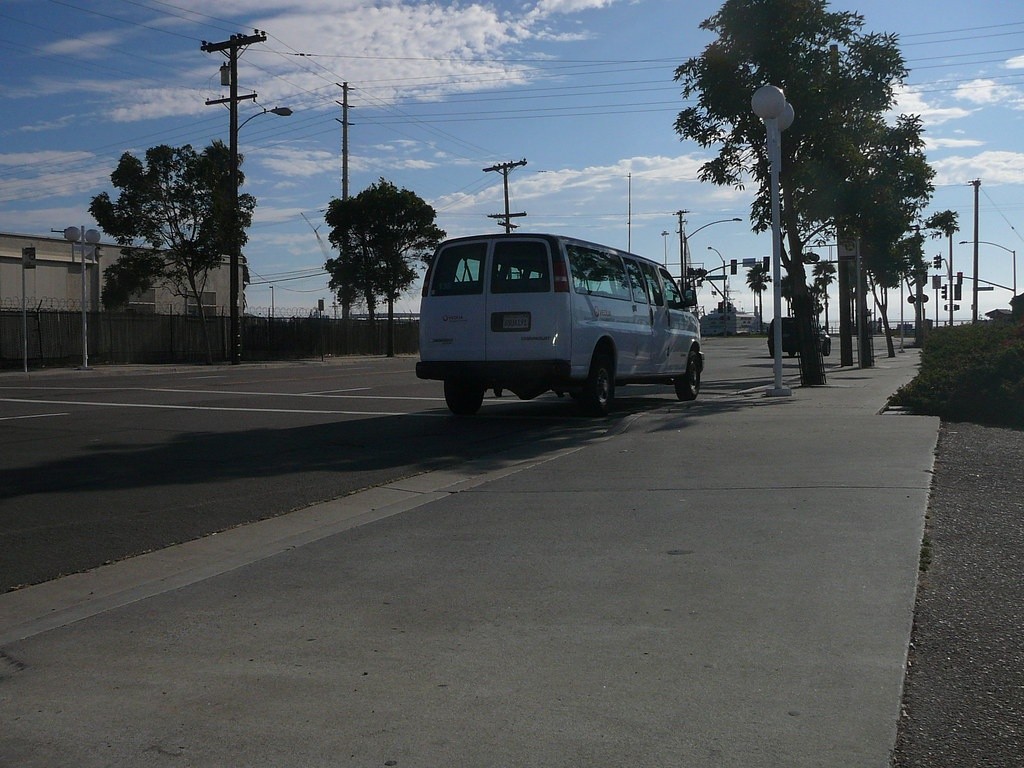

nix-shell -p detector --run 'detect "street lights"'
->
[752,85,796,396]
[959,241,1017,325]
[64,226,102,370]
[683,217,742,300]
[708,246,728,337]
[661,230,670,270]
[228,104,293,364]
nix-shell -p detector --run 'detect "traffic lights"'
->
[934,255,938,269]
[941,284,946,300]
[937,255,942,269]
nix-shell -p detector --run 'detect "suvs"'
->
[415,234,705,419]
